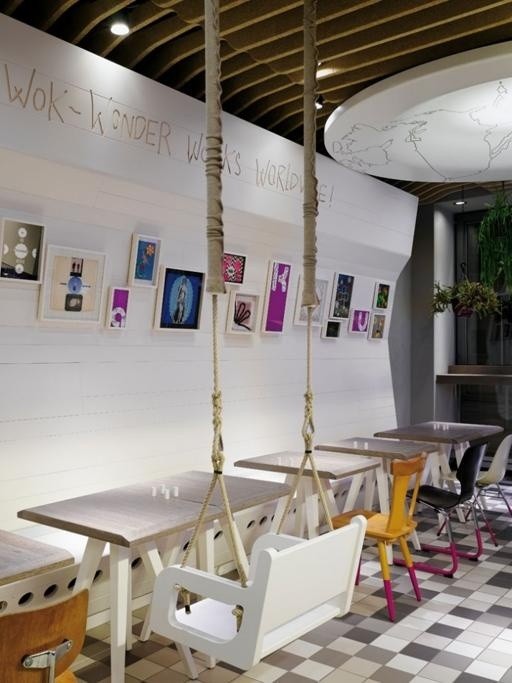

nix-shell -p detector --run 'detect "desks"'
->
[1,527,77,590]
[368,419,504,536]
[234,447,383,544]
[18,469,296,682]
[314,434,443,566]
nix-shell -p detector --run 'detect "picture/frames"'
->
[348,306,374,334]
[321,318,343,340]
[36,243,109,325]
[372,281,392,311]
[367,312,388,342]
[150,264,209,332]
[291,272,332,328]
[224,251,249,286]
[126,231,164,289]
[103,286,131,329]
[260,258,293,335]
[224,288,264,336]
[328,271,358,321]
[0,215,48,285]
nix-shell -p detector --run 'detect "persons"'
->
[176,277,187,323]
[378,285,388,307]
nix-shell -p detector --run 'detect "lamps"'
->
[324,39,512,186]
[316,93,325,110]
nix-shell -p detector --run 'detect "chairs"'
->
[149,514,369,673]
[431,431,512,548]
[331,447,432,624]
[0,588,97,683]
[389,441,489,576]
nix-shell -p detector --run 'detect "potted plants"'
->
[428,262,502,321]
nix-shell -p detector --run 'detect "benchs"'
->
[2,452,429,631]
